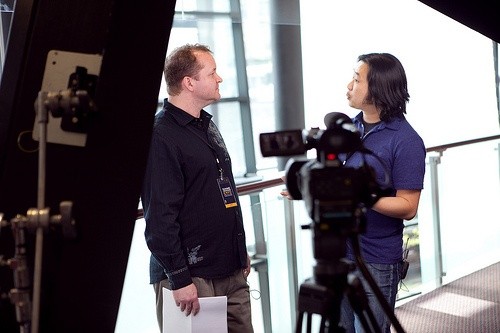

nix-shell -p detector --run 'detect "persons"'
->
[140,45,254,333]
[280,53,426,333]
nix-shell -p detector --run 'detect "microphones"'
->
[324,112,360,133]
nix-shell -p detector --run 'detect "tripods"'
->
[296,223,384,333]
